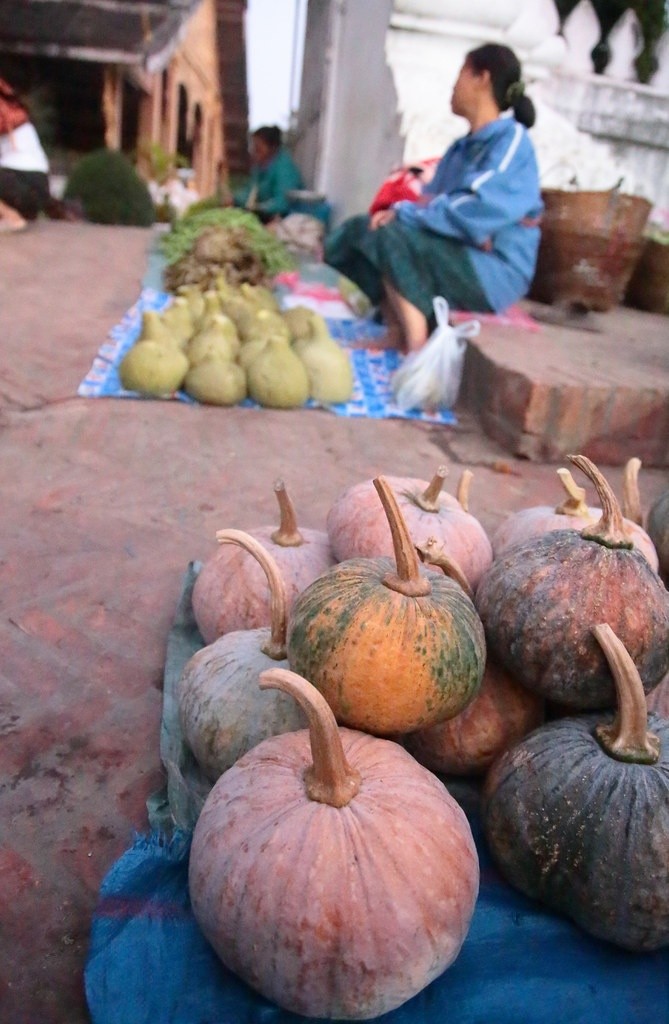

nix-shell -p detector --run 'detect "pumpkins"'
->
[180,451,669,1023]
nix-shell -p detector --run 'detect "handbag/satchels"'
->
[388,295,481,412]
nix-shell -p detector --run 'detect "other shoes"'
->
[0,215,28,236]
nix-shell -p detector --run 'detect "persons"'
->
[316,39,547,365]
[233,125,303,229]
[1,80,52,233]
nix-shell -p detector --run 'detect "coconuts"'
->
[119,280,354,408]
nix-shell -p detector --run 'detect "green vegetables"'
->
[166,194,294,293]
